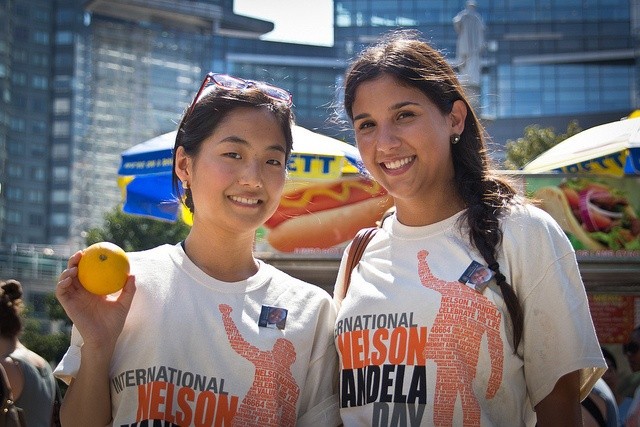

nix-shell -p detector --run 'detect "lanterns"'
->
[78,240,131,295]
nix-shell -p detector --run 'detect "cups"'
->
[185,72,294,126]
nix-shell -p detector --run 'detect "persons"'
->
[580,324,640,427]
[53,71,338,427]
[331,36,609,427]
[459,258,493,293]
[258,305,288,328]
[1,279,57,427]
